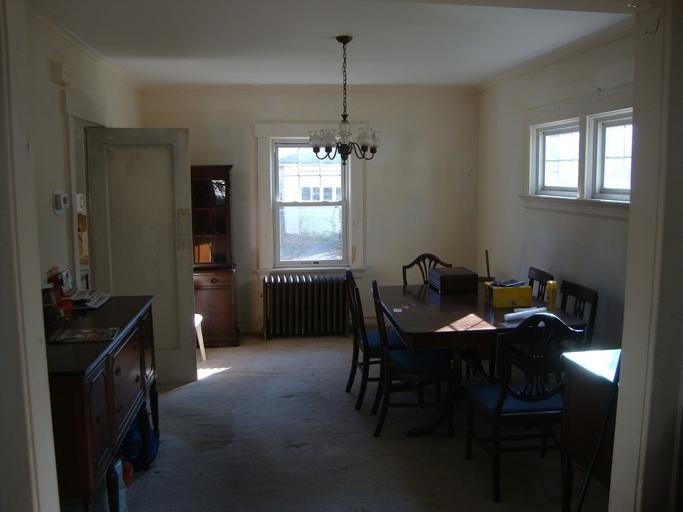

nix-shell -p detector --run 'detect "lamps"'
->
[307,36,382,164]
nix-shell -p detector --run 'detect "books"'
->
[60,286,112,309]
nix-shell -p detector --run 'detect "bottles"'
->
[547,280,557,314]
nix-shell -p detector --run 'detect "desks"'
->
[372,279,587,446]
[558,348,621,511]
[43,296,159,510]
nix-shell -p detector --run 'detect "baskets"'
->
[428,267,478,294]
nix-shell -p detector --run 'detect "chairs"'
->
[398,256,456,287]
[526,264,557,299]
[368,278,464,439]
[344,266,439,415]
[497,279,596,419]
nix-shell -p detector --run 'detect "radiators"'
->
[262,274,349,340]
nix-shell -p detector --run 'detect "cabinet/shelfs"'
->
[190,164,239,347]
